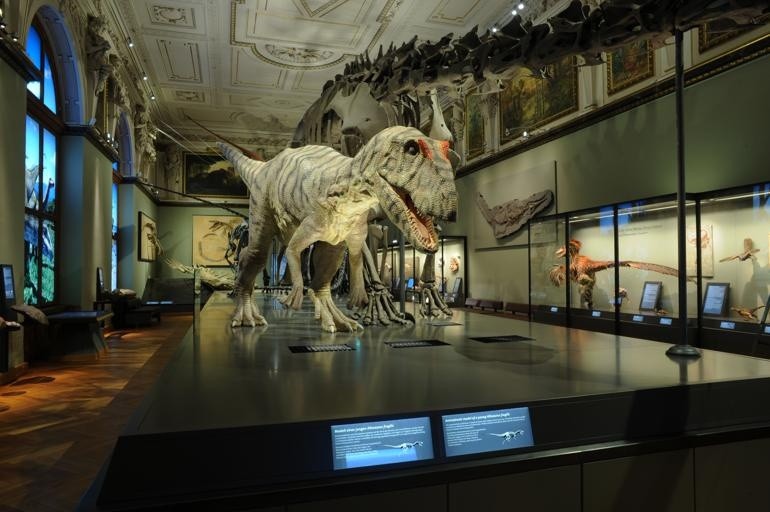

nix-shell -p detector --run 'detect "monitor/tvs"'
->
[0,264,16,306]
[97,268,104,287]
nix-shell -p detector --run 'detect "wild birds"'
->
[719,238,761,263]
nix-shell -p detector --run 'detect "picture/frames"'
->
[191,214,248,269]
[703,282,730,317]
[181,150,251,199]
[498,56,579,146]
[638,281,662,311]
[697,1,768,55]
[464,95,486,162]
[138,211,159,261]
[604,34,656,95]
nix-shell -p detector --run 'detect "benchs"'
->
[43,311,108,360]
[129,306,162,328]
